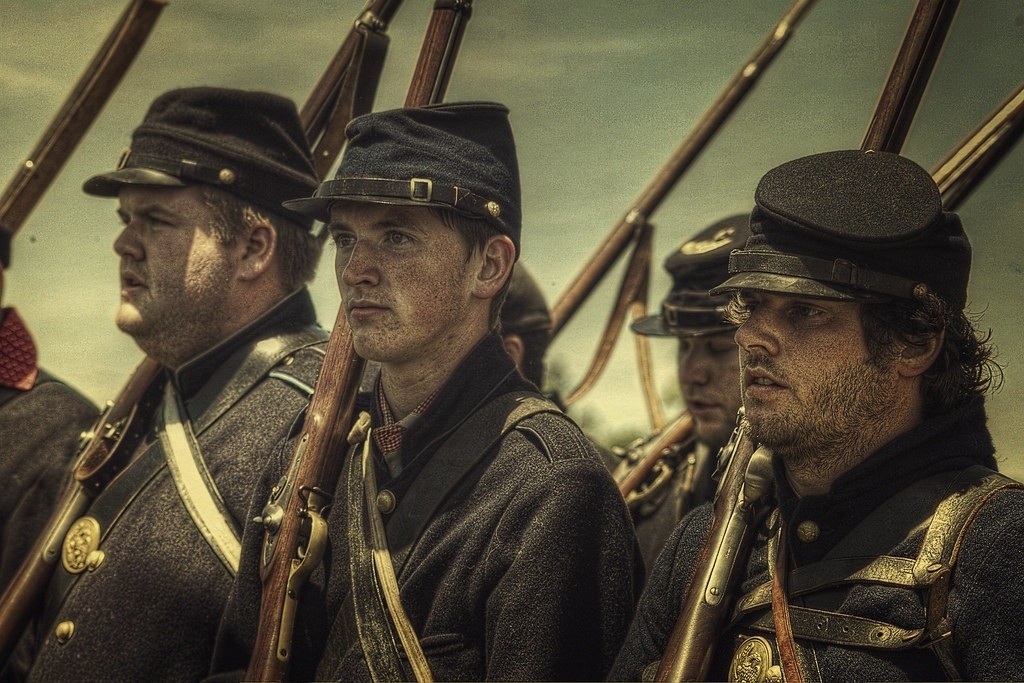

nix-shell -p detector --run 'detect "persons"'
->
[1,224,100,593]
[607,149,1024,683]
[235,102,646,683]
[0,85,380,683]
[612,213,749,580]
[499,260,622,471]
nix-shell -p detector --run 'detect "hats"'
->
[628,214,759,337]
[708,151,971,310]
[82,87,320,231]
[281,101,521,263]
[500,264,551,334]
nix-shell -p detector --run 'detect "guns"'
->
[612,86,1024,507]
[243,0,487,683]
[640,0,960,683]
[0,0,407,683]
[542,0,821,347]
